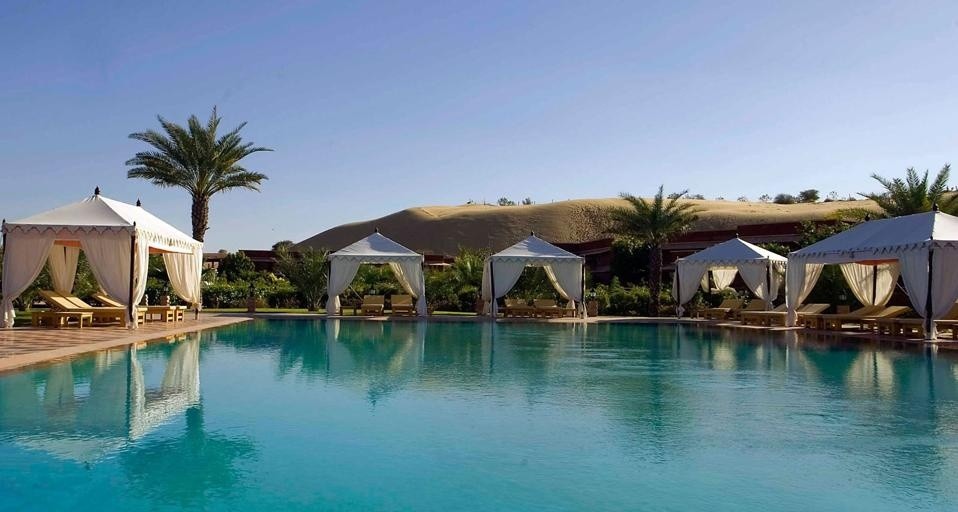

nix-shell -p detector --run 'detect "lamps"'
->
[163,281,168,296]
[591,288,597,301]
[478,287,482,299]
[248,283,255,297]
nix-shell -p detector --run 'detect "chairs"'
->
[30,286,188,329]
[391,294,414,316]
[361,295,385,315]
[534,298,561,317]
[691,298,958,340]
[504,298,535,317]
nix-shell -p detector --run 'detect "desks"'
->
[561,309,577,317]
[340,306,357,316]
